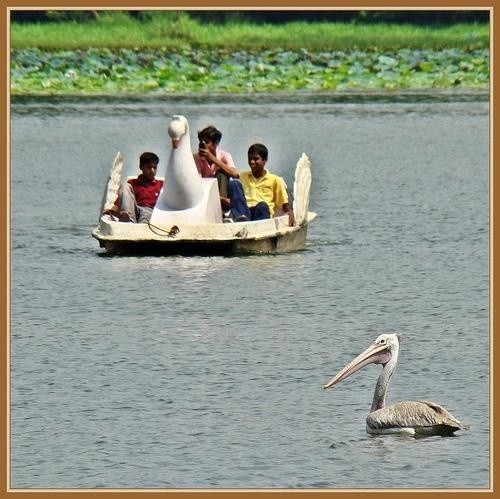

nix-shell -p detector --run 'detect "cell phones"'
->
[199,143,204,155]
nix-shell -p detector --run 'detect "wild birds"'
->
[322,331,471,438]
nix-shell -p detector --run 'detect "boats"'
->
[91,112,322,257]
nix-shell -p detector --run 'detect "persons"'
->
[220,143,297,227]
[101,152,164,225]
[193,125,240,198]
